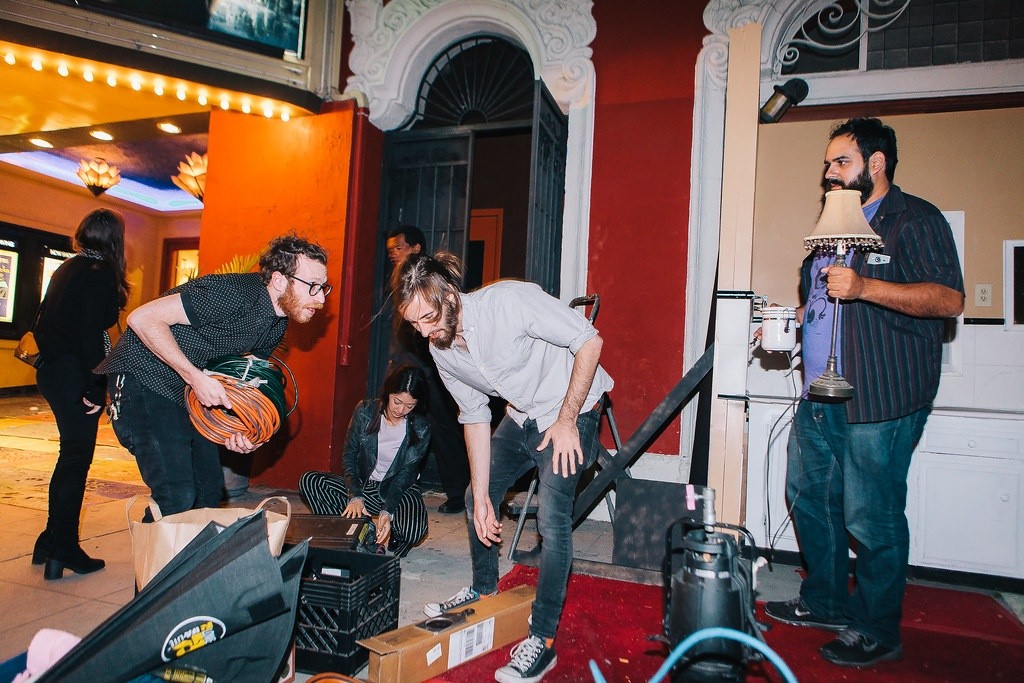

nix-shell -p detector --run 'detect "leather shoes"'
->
[438,497,466,512]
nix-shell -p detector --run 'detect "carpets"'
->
[401,568,1022,683]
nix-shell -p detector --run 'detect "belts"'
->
[594,402,603,414]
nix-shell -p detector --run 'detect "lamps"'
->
[802,190,886,398]
[78,157,123,197]
[171,149,205,205]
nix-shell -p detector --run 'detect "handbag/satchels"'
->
[14,300,44,368]
[125,494,291,593]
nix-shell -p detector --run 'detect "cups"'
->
[761,307,797,351]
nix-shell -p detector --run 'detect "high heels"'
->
[31,530,48,564]
[43,536,106,580]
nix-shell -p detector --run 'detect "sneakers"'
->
[422,588,498,618]
[818,630,903,668]
[763,597,848,633]
[495,633,558,683]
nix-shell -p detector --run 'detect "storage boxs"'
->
[281,545,400,678]
[354,584,540,683]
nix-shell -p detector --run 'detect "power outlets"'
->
[974,283,993,307]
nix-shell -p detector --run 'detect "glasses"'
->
[285,273,333,297]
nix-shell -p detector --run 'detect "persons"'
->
[298,362,432,561]
[754,120,967,670]
[92,227,330,599]
[375,225,468,514]
[386,249,615,683]
[32,210,132,579]
